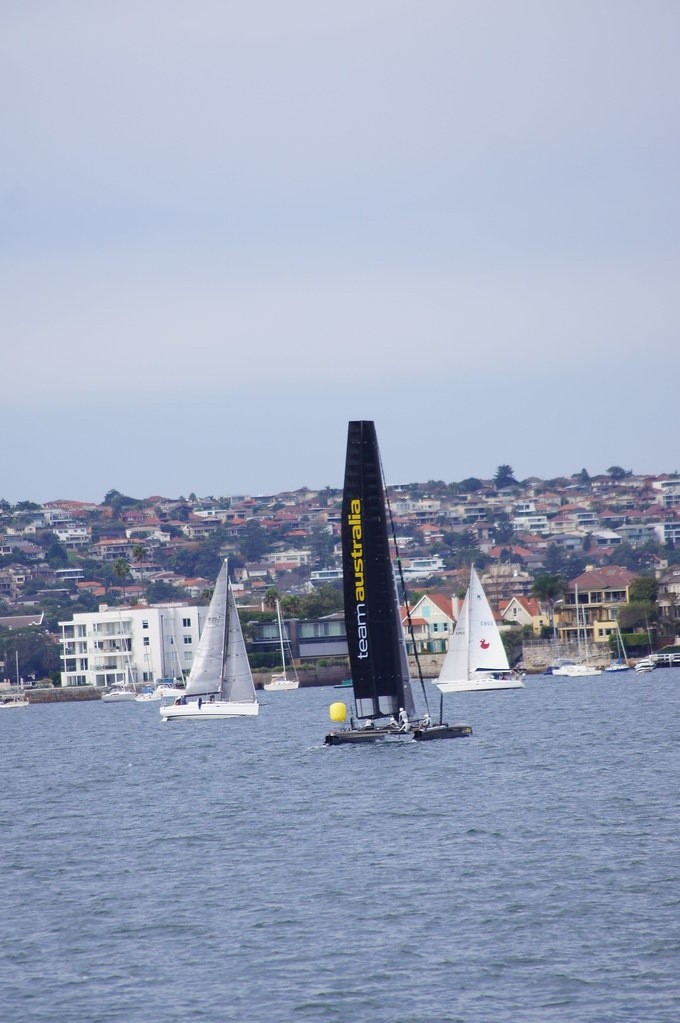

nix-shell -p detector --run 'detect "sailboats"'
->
[264,598,300,691]
[432,561,526,694]
[158,557,260,719]
[323,420,472,745]
[0,651,30,708]
[101,608,186,704]
[544,583,631,676]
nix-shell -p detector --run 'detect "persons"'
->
[174,694,215,710]
[387,708,412,734]
[419,714,432,730]
[365,717,375,730]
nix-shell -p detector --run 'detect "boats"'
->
[635,659,656,673]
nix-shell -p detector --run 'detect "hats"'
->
[404,719,408,723]
[424,714,429,717]
[399,708,403,711]
